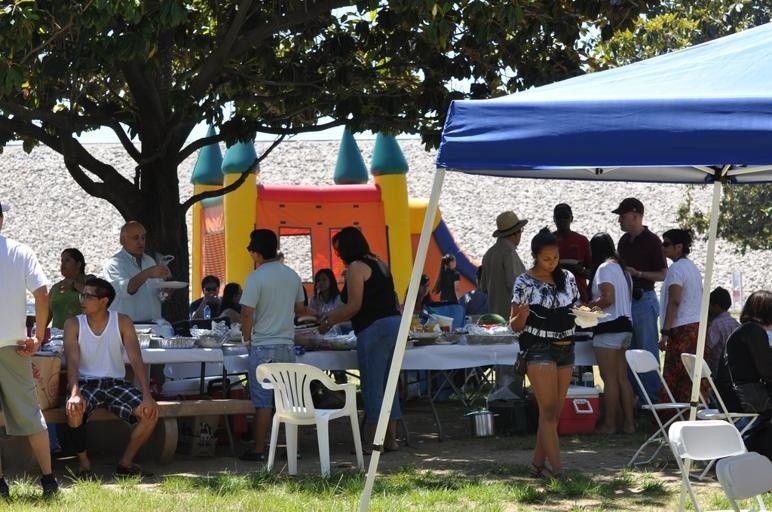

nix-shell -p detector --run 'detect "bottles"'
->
[202,304,211,319]
[30,322,37,338]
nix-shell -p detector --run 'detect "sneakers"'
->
[0,477,8,496]
[116,464,153,477]
[41,477,58,495]
[240,452,266,461]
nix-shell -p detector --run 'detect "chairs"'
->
[625,349,772,512]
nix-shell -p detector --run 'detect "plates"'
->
[154,281,187,289]
[572,309,609,318]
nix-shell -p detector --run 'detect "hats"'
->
[493,212,528,237]
[612,198,643,214]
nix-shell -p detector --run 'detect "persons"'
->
[0,202,65,499]
[46,195,772,484]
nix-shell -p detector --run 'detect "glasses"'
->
[204,287,217,291]
[79,293,97,299]
[662,242,672,246]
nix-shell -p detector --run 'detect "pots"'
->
[463,407,500,438]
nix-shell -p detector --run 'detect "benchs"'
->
[1,399,257,465]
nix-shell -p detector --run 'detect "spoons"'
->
[528,309,546,319]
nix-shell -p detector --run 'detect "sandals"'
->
[529,463,552,478]
